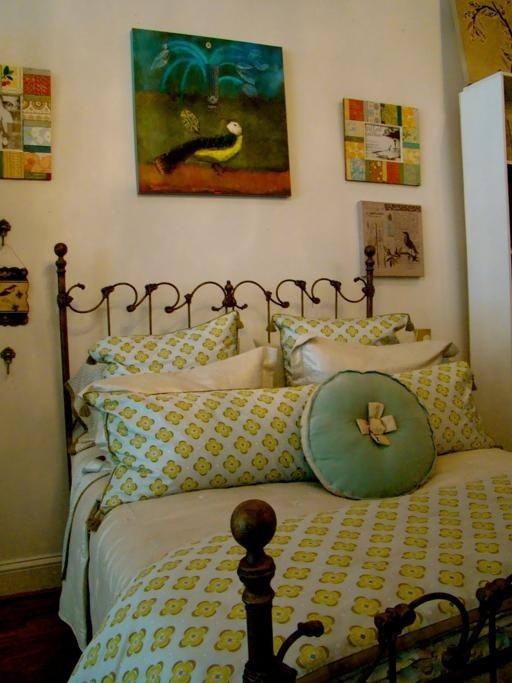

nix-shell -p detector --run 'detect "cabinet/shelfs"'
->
[457,72,512,450]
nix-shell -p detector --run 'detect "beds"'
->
[54,242,512,682]
